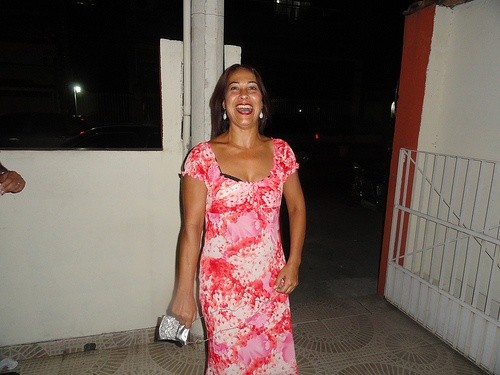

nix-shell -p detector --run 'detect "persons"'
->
[171,64,306,375]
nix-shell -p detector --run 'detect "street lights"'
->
[73,85,82,116]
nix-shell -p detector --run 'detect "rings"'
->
[17,182,21,185]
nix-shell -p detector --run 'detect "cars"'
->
[264,109,321,164]
[0,112,89,148]
[59,124,162,148]
